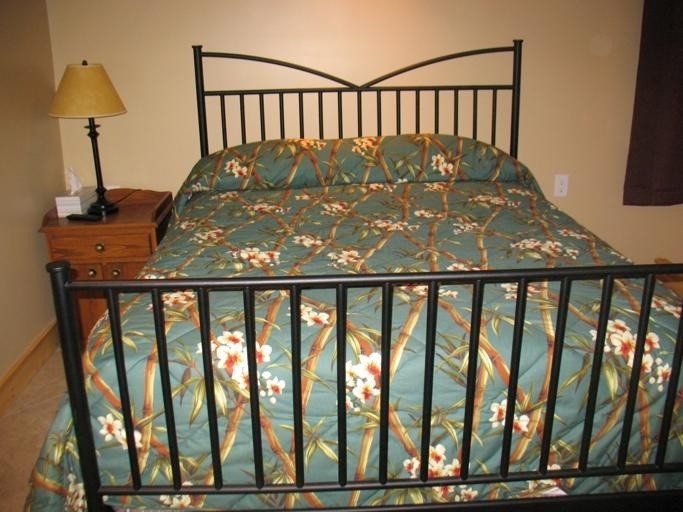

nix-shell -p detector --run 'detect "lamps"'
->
[50,59,129,221]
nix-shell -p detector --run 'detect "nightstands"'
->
[39,188,173,356]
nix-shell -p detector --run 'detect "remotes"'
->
[65,213,103,222]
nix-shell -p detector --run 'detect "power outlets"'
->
[557,177,570,200]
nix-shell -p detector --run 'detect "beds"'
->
[24,38,683,511]
